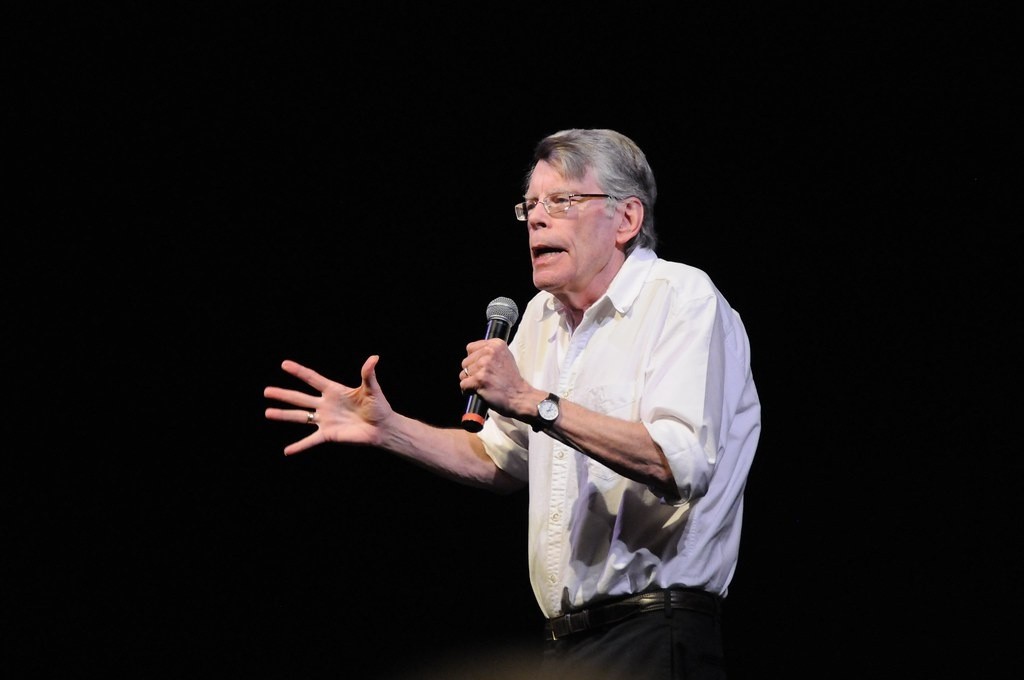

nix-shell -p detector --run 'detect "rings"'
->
[306,411,314,424]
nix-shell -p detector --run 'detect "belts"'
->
[543,589,706,641]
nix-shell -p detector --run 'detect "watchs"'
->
[532,393,560,433]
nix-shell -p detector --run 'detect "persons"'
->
[263,129,762,680]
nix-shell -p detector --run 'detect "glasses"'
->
[514,193,611,222]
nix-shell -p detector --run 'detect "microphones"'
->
[461,296,519,433]
